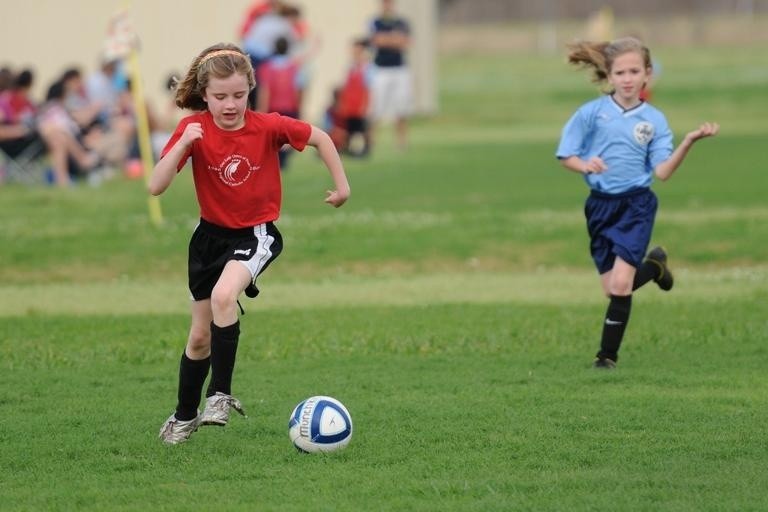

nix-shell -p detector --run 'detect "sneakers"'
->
[158,407,202,447]
[644,245,674,292]
[590,349,618,370]
[200,391,231,427]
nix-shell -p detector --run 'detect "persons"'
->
[555,38,722,372]
[241,2,312,169]
[0,50,173,185]
[145,42,350,447]
[325,1,420,155]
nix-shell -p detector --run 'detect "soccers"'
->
[288,396,353,455]
